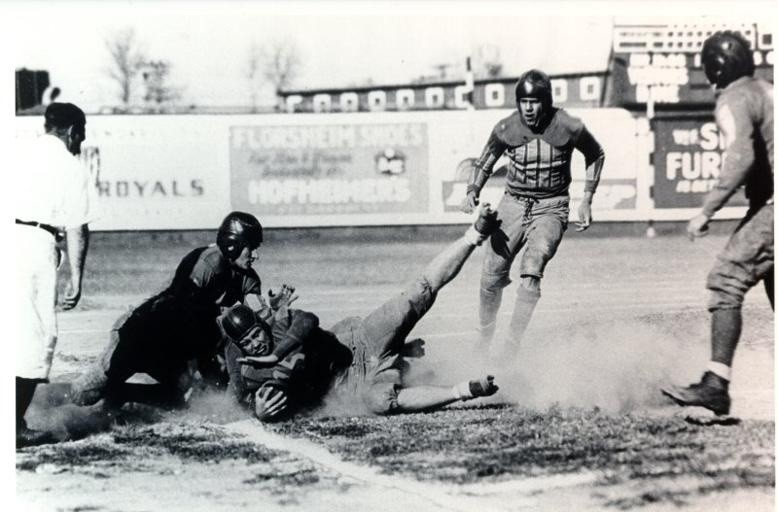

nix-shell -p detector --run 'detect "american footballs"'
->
[259,384,295,423]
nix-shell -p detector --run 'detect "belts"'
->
[16,219,56,236]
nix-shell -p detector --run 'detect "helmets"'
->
[702,33,756,88]
[515,70,552,126]
[223,305,272,359]
[217,212,263,259]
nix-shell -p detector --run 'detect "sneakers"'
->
[17,428,46,449]
[467,374,498,398]
[471,204,500,242]
[661,383,731,416]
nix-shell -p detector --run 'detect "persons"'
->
[463,70,605,364]
[221,202,499,421]
[30,211,298,413]
[661,33,774,414]
[16,102,93,446]
